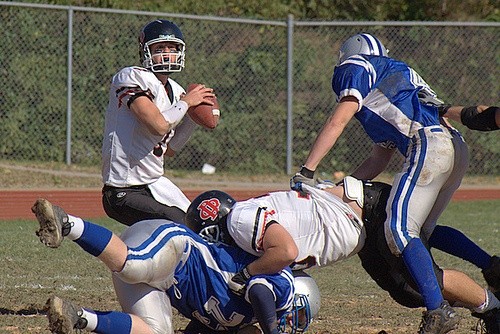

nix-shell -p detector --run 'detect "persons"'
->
[417,85,500,132]
[185,176,500,334]
[101,19,216,225]
[288,33,468,334]
[31,198,320,334]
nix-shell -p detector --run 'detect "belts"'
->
[175,241,189,275]
[430,128,461,137]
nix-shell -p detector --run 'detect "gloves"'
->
[418,87,453,117]
[317,176,344,186]
[290,167,316,192]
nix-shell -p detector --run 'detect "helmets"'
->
[288,270,321,333]
[185,190,236,246]
[138,19,187,75]
[337,34,388,64]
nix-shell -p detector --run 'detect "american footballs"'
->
[186,83,220,129]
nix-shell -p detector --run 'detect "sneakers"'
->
[480,256,500,301]
[45,295,89,334]
[32,197,75,249]
[470,295,500,334]
[418,300,459,334]
